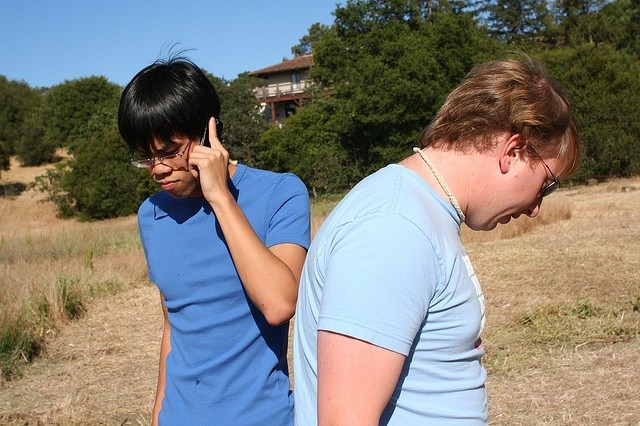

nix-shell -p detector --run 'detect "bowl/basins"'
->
[412,145,466,223]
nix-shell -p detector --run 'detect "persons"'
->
[293,43,584,425]
[116,40,311,425]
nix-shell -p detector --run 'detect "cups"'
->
[129,139,192,168]
[510,123,561,200]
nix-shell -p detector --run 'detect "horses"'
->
[200,118,223,147]
